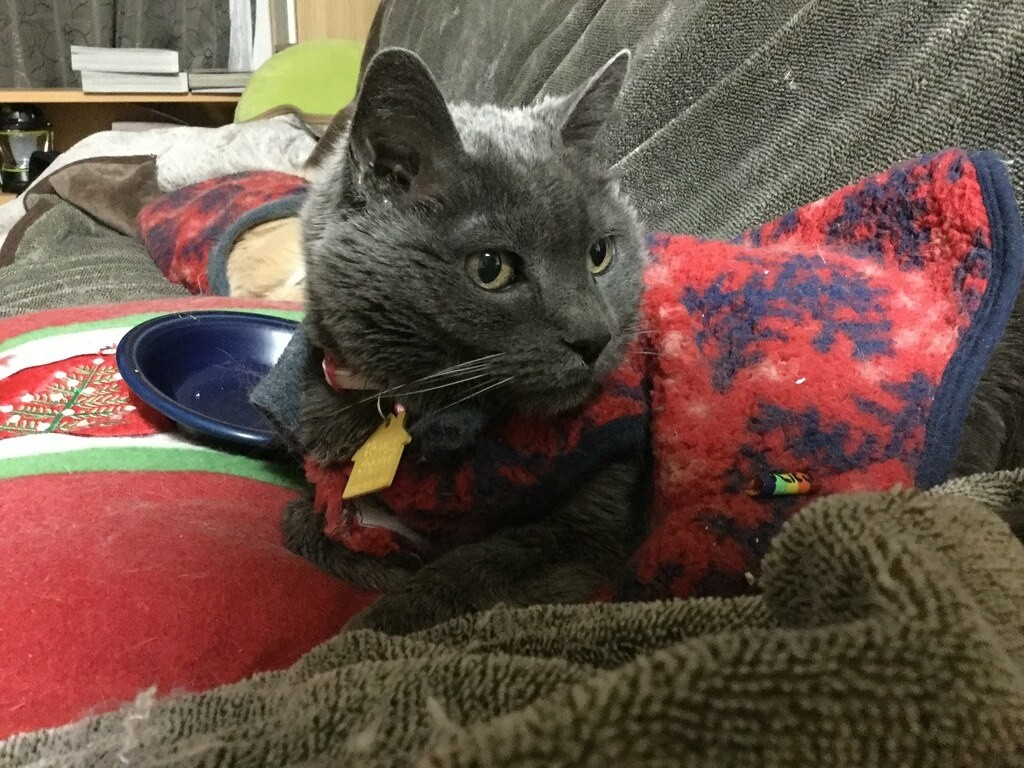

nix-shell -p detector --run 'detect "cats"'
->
[279,47,1024,638]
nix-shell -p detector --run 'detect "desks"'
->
[0,87,241,153]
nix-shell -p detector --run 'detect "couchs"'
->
[0,0,1023,768]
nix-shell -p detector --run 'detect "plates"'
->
[117,312,300,449]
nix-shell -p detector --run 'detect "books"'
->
[70,45,255,93]
